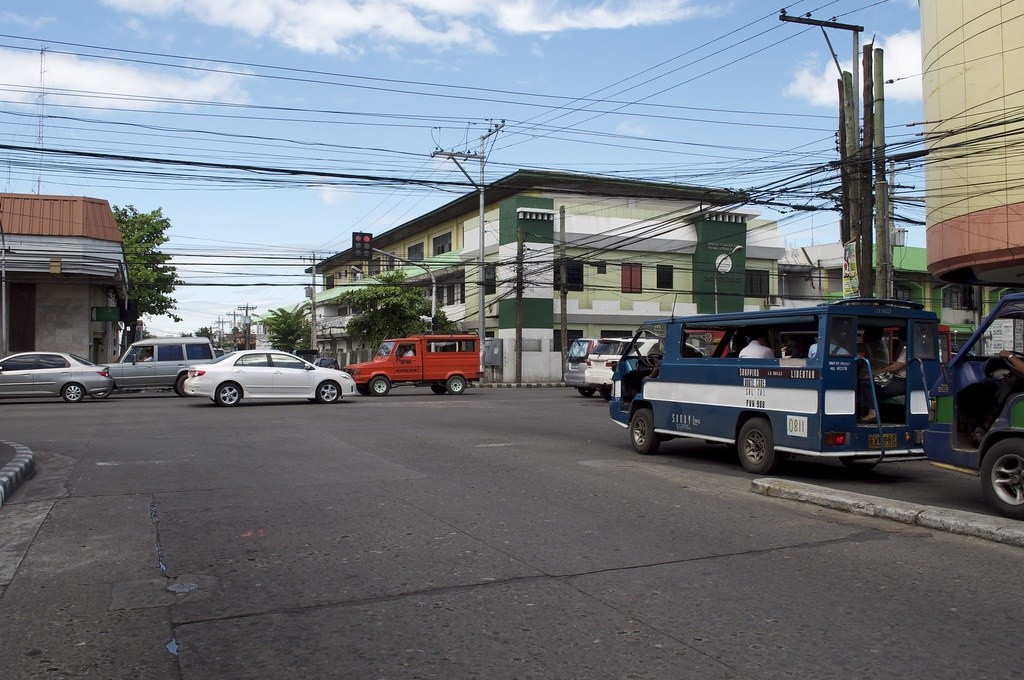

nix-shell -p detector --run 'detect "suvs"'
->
[344,332,484,396]
[585,337,662,401]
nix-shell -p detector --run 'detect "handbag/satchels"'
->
[873,366,893,388]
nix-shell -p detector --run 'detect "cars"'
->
[313,357,339,369]
[0,352,112,402]
[183,350,356,407]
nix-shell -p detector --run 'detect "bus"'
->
[609,298,940,474]
[921,289,1024,520]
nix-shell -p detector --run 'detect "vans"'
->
[563,338,603,397]
[98,337,217,397]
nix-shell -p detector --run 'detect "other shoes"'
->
[860,417,877,424]
[975,427,985,443]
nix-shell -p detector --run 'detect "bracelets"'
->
[1008,353,1014,359]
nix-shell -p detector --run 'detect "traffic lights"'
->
[351,232,373,260]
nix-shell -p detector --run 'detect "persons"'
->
[739,326,776,358]
[976,350,1024,439]
[643,332,702,381]
[863,326,889,374]
[781,334,850,358]
[724,334,748,358]
[398,345,414,356]
[861,326,907,423]
[136,349,153,361]
